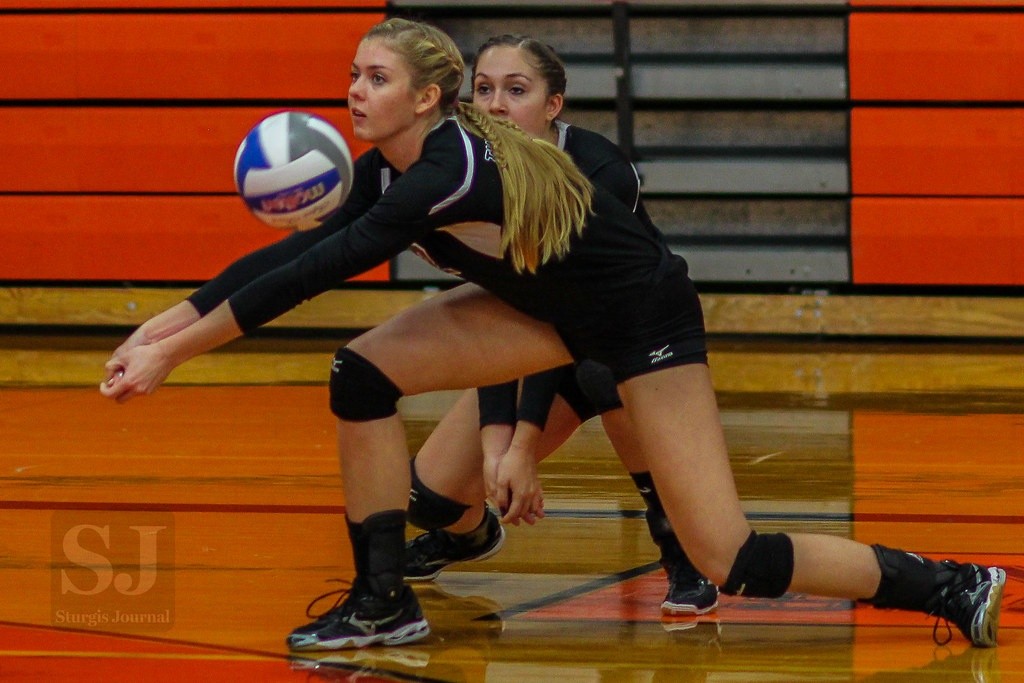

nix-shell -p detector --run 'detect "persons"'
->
[100,17,1008,651]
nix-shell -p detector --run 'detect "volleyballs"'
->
[234,110,359,233]
